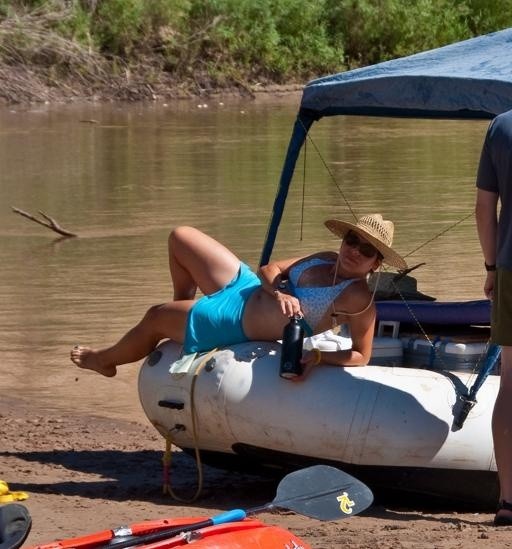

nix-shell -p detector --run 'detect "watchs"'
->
[272,281,290,297]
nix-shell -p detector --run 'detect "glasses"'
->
[343,232,385,258]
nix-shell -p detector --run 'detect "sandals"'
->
[492,499,512,526]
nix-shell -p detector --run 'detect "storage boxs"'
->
[368,335,501,375]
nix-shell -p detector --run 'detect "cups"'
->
[278,314,304,381]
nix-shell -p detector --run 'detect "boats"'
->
[138,332,503,503]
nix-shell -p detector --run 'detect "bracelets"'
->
[310,346,323,365]
[483,260,497,272]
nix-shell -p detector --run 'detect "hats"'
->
[323,212,410,270]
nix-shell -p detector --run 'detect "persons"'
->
[473,105,512,526]
[70,202,407,383]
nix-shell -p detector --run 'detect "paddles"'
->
[72,465,373,548]
